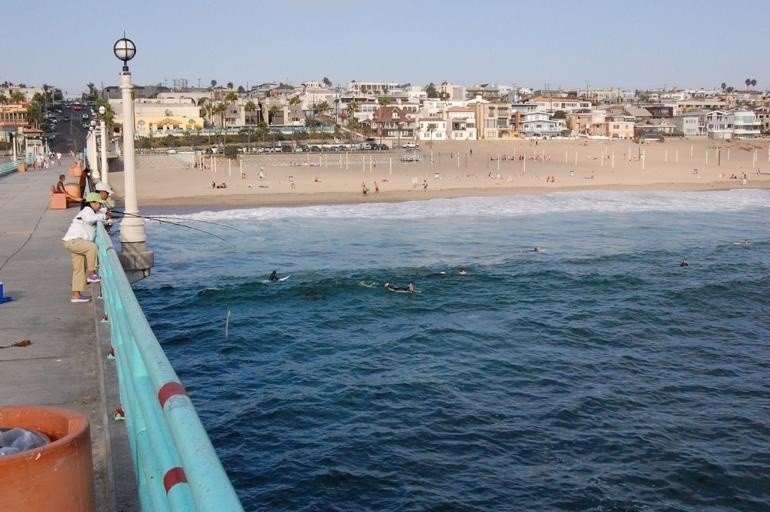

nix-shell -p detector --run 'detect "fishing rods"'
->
[110,210,245,247]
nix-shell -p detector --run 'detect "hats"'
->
[96,182,114,195]
[86,193,105,204]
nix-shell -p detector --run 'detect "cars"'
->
[401,142,420,148]
[201,142,389,155]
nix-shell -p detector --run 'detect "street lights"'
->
[85,104,112,192]
[113,28,148,243]
[11,131,48,164]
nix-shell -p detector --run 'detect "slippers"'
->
[71,295,90,302]
[86,274,101,283]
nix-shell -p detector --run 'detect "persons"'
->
[60,192,104,303]
[269,270,279,281]
[362,185,367,195]
[408,281,414,291]
[680,258,688,267]
[56,175,83,202]
[79,169,92,197]
[95,182,113,219]
[32,152,61,170]
[374,181,379,192]
